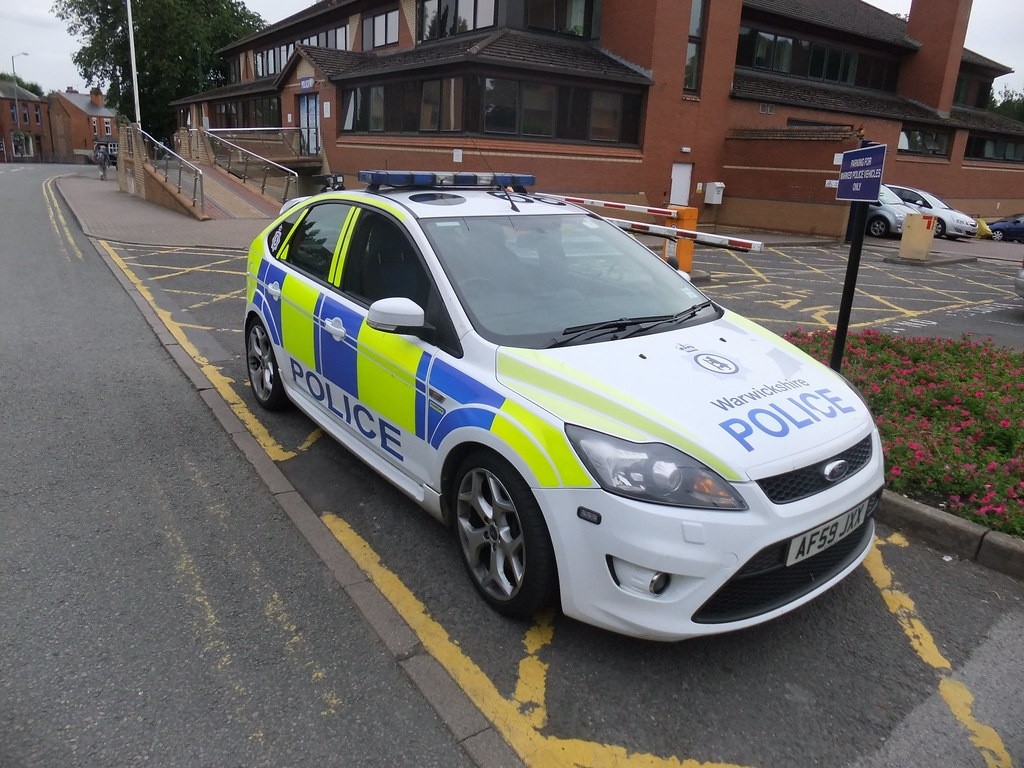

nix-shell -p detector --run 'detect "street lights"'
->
[11,52,29,128]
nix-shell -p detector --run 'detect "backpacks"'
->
[97,152,105,165]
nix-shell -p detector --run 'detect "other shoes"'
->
[100,175,103,181]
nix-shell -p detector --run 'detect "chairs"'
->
[364,222,430,314]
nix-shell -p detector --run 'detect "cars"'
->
[865,184,921,237]
[109,151,118,167]
[987,213,1024,241]
[243,170,885,643]
[885,183,978,238]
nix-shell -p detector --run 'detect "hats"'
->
[100,146,106,149]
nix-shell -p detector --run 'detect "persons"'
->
[95,145,111,181]
